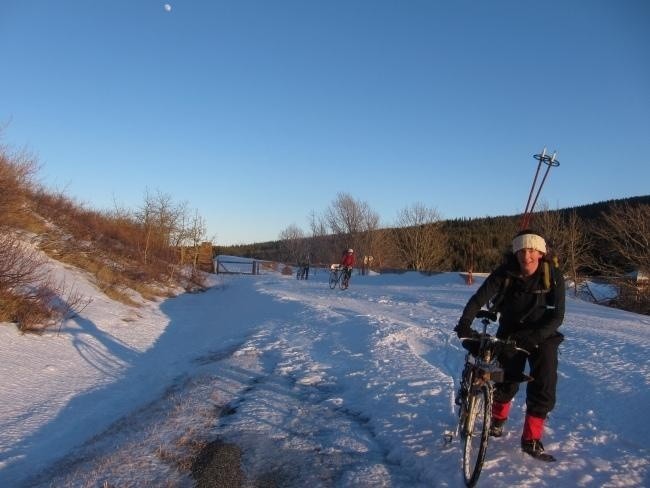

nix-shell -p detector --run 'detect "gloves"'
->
[457,319,480,356]
[513,338,536,360]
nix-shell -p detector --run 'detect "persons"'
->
[339,248,355,290]
[300,254,310,281]
[467,271,473,286]
[457,227,566,461]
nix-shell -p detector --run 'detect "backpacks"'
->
[342,248,348,258]
[489,249,559,315]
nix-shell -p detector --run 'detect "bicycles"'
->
[296,265,307,281]
[329,264,353,290]
[442,310,532,488]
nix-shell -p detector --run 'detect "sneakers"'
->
[488,415,509,437]
[521,435,557,462]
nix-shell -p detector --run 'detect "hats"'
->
[511,229,547,256]
[348,248,353,253]
